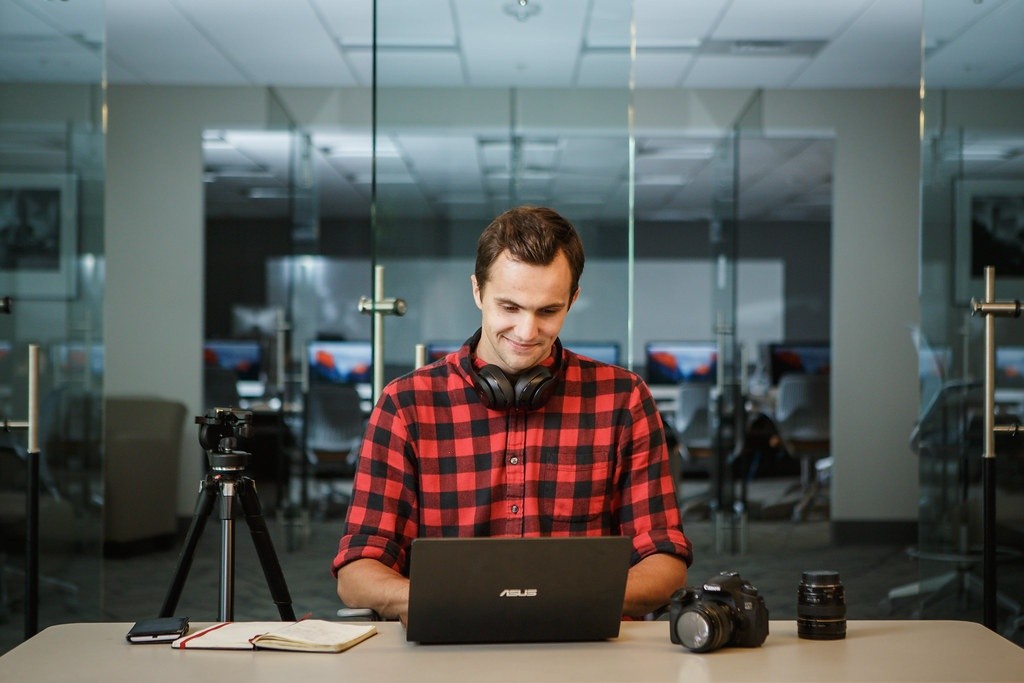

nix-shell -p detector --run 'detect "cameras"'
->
[669,570,770,653]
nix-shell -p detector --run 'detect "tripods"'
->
[157,448,298,622]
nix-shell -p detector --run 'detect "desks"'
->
[0,619,1024,683]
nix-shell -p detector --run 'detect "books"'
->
[126,616,377,654]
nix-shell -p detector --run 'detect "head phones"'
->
[466,324,566,412]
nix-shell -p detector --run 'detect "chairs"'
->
[675,375,829,526]
[285,373,368,515]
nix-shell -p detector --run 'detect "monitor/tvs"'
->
[0,340,1024,393]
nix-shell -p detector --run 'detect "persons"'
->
[331,204,700,632]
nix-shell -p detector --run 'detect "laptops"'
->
[405,535,632,642]
